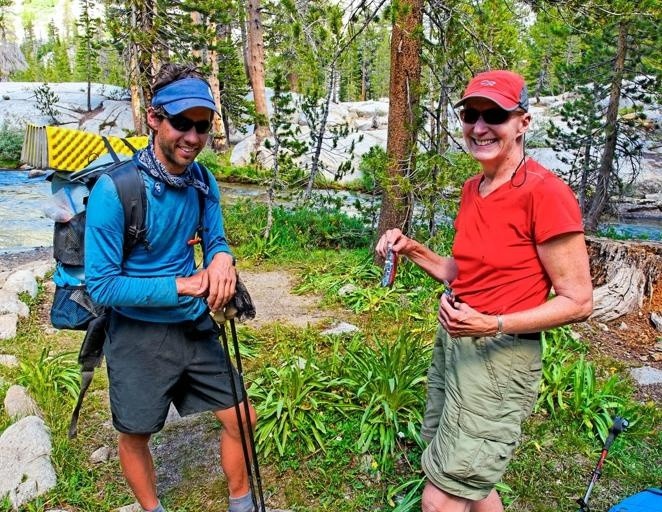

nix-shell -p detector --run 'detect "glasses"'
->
[459,107,510,125]
[167,114,213,133]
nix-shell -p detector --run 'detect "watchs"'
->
[493,314,503,340]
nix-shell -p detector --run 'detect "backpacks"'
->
[44,153,210,331]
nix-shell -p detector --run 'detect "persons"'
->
[83,63,296,512]
[375,69,593,512]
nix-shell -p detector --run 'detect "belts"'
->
[446,295,541,340]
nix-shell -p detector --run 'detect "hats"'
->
[151,78,221,117]
[453,69,529,113]
